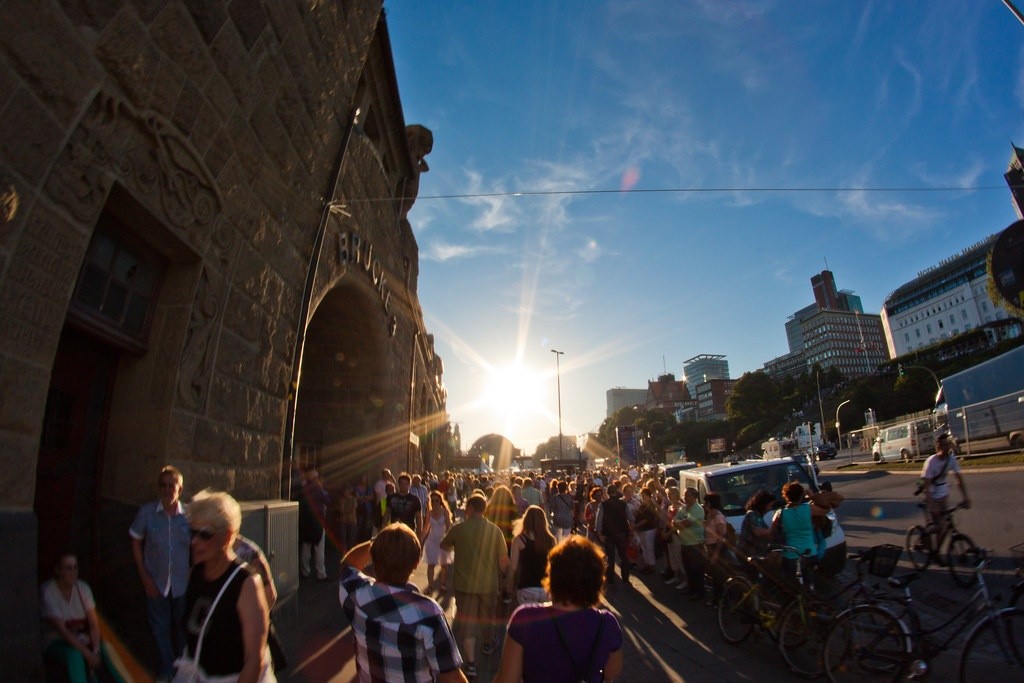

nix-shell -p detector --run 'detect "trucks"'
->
[928,344,1023,460]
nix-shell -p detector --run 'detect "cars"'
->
[805,444,838,462]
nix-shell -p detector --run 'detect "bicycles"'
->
[906,504,981,590]
[764,542,1023,683]
[717,542,854,678]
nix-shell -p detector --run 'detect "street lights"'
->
[551,349,565,458]
[836,399,850,451]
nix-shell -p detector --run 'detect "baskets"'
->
[864,544,903,577]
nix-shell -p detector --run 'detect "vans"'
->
[656,462,698,487]
[871,414,938,465]
[761,438,799,460]
[677,456,848,576]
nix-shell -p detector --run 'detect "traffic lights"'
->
[898,363,905,378]
[809,422,816,435]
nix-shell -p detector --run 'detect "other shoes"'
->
[609,560,728,610]
[313,572,331,584]
[464,662,478,677]
[423,584,433,594]
[481,635,499,654]
[441,581,447,592]
[302,574,312,587]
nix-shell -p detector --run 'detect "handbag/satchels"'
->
[65,618,93,647]
[170,648,278,683]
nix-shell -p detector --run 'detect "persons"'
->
[548,463,727,610]
[43,555,114,683]
[737,489,778,617]
[282,466,550,595]
[168,491,279,683]
[721,455,743,462]
[439,494,511,673]
[918,433,970,556]
[129,465,198,683]
[768,484,831,606]
[337,523,469,683]
[492,537,622,683]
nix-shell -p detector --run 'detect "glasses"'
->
[187,527,221,540]
[432,499,440,503]
